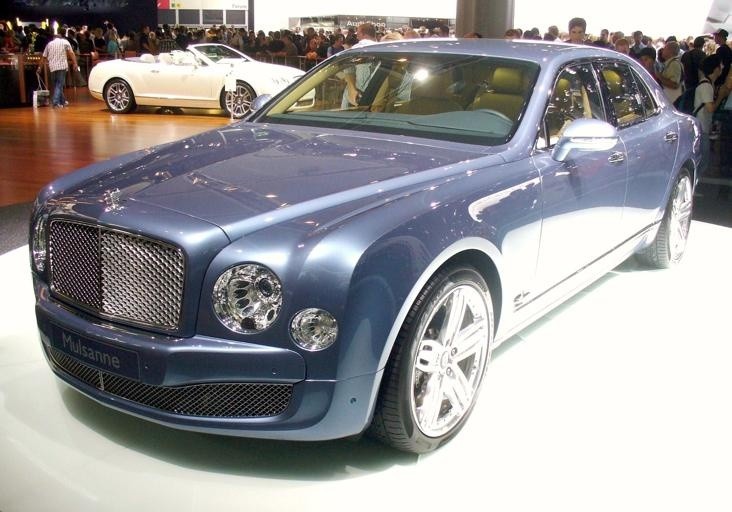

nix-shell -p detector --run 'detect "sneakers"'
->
[52,100,70,110]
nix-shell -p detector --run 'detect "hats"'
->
[710,28,729,40]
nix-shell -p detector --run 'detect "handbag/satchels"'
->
[33,89,50,108]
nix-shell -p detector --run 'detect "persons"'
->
[460,16,732,211]
[0,15,457,113]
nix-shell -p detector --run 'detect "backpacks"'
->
[673,78,710,119]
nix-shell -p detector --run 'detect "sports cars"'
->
[86,42,316,116]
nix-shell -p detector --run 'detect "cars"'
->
[28,37,703,459]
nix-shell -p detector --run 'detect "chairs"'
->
[394,62,633,137]
[141,50,195,65]
